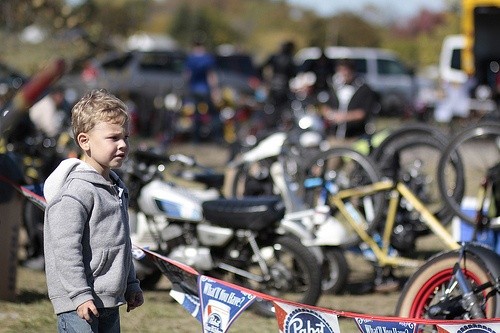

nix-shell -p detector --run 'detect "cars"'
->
[0,0,500,129]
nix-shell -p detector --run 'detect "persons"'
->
[186,40,379,142]
[43,90,144,333]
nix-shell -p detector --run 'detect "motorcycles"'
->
[1,110,500,333]
